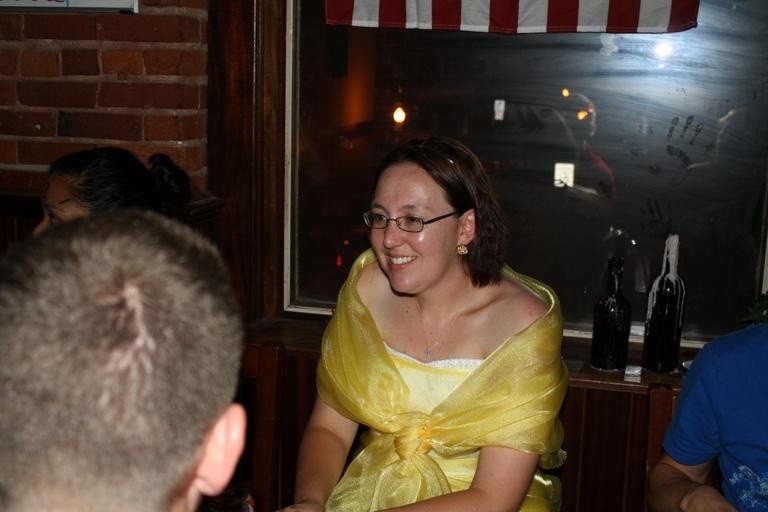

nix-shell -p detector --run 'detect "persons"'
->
[272,133,573,512]
[641,318,767,512]
[0,202,251,512]
[507,93,618,325]
[26,144,191,246]
[646,107,765,325]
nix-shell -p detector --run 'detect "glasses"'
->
[360,204,459,232]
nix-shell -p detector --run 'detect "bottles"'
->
[590,234,686,376]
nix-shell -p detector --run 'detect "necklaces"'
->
[407,306,465,363]
